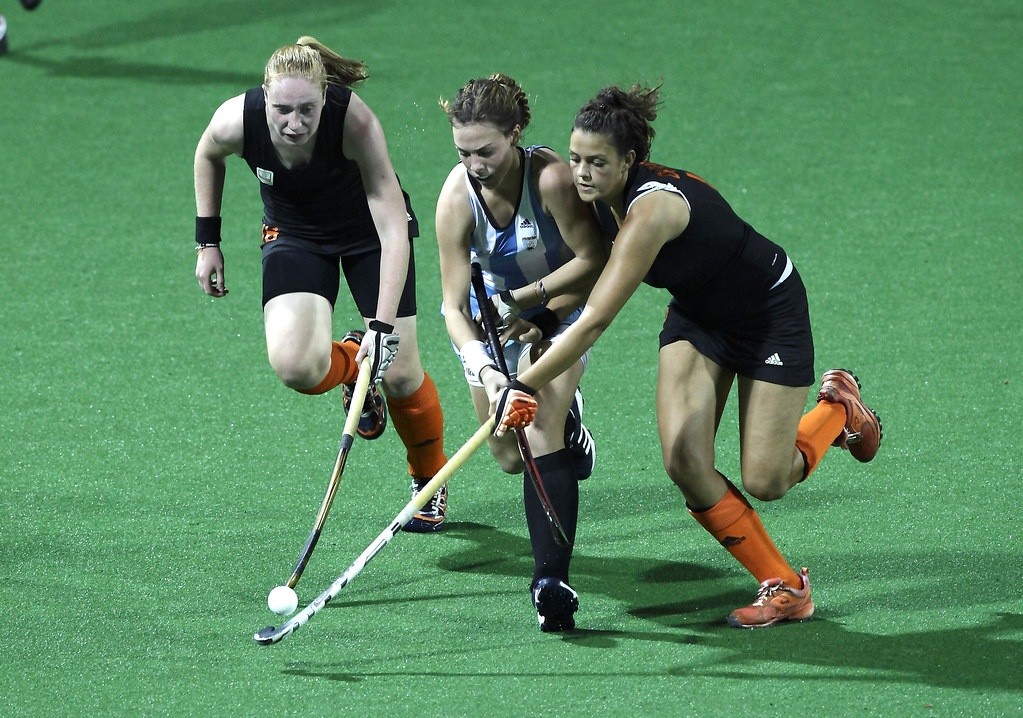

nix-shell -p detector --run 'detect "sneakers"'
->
[341,330,388,441]
[817,368,883,463]
[567,387,598,483]
[729,566,814,628]
[403,478,449,531]
[534,577,578,631]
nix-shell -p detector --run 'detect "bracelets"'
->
[194,242,220,255]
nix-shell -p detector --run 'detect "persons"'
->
[480,73,881,630]
[435,72,614,632]
[193,35,450,530]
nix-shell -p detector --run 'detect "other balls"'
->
[269,585,298,615]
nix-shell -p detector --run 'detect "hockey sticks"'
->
[465,248,576,551]
[284,355,383,590]
[252,414,504,647]
